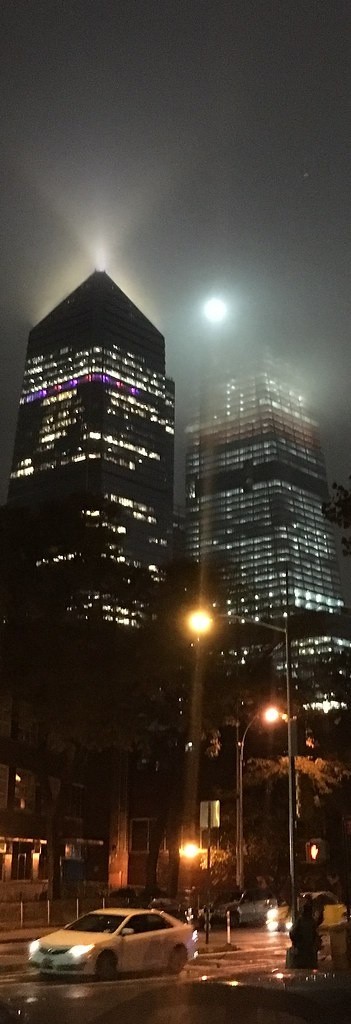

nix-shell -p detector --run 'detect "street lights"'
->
[188,609,298,931]
[237,699,280,894]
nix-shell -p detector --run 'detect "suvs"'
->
[201,888,279,928]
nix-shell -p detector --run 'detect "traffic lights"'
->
[307,839,325,865]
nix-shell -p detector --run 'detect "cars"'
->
[282,891,342,923]
[28,907,198,982]
